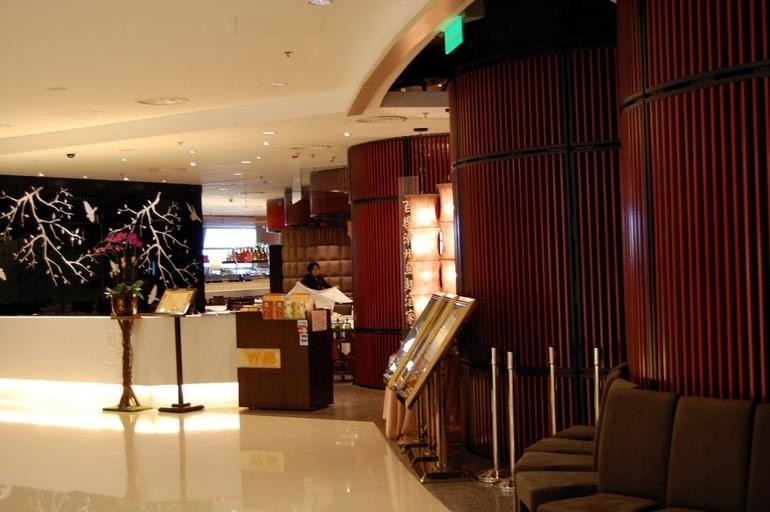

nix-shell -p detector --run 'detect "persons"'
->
[298,261,340,290]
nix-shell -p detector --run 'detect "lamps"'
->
[291,175,301,204]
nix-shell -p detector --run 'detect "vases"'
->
[111,293,138,317]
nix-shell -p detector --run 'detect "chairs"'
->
[204,294,268,311]
[511,363,770,512]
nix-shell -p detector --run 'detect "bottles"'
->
[345,319,351,333]
[230,246,267,261]
[335,318,342,340]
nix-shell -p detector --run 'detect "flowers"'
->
[95,232,144,299]
